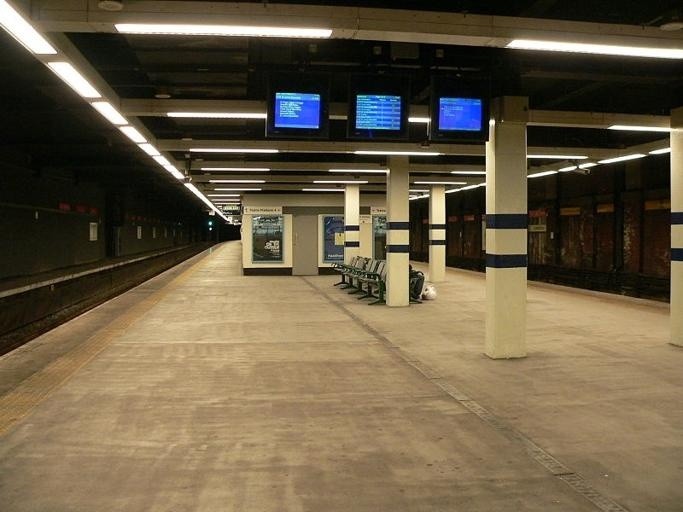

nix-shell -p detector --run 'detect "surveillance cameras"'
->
[183,175,194,183]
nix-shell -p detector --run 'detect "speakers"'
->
[499,96,531,125]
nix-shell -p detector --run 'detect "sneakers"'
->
[410,298,422,304]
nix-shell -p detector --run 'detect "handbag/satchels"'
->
[422,287,436,299]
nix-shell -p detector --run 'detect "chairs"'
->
[331,256,425,305]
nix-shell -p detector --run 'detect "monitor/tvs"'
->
[348,92,409,141]
[264,86,329,141]
[430,92,488,143]
